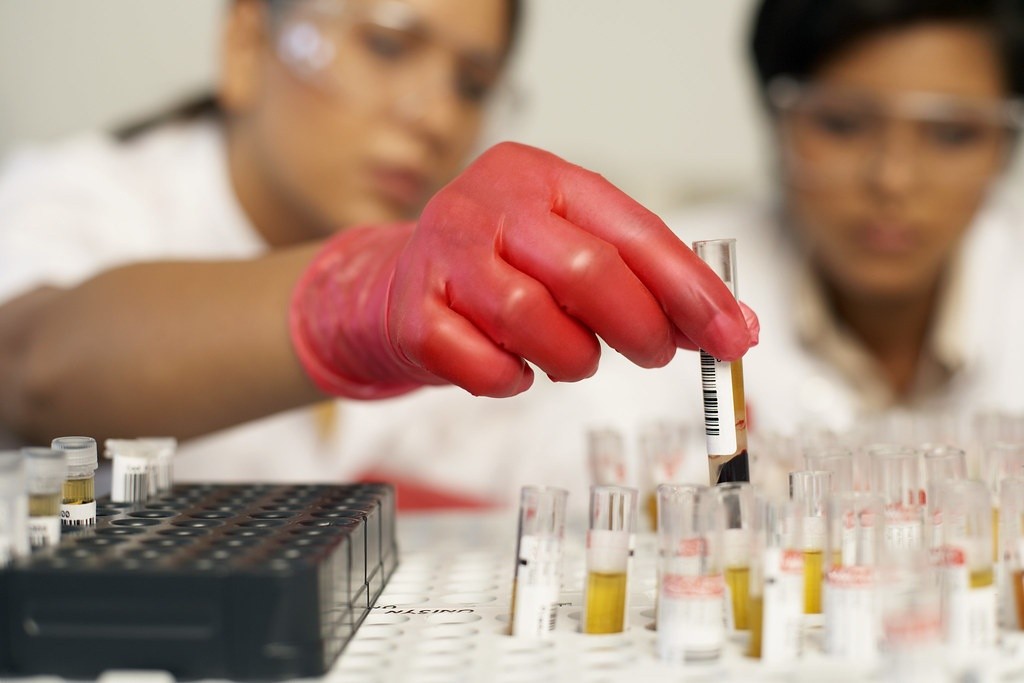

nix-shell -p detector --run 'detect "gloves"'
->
[290,139,761,400]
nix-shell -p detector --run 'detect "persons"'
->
[0,1,760,511]
[590,1,1023,489]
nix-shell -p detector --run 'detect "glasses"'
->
[267,0,526,151]
[767,76,1024,210]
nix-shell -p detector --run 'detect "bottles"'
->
[511,419,1023,683]
[106,436,176,502]
[692,238,752,484]
[0,436,97,565]
